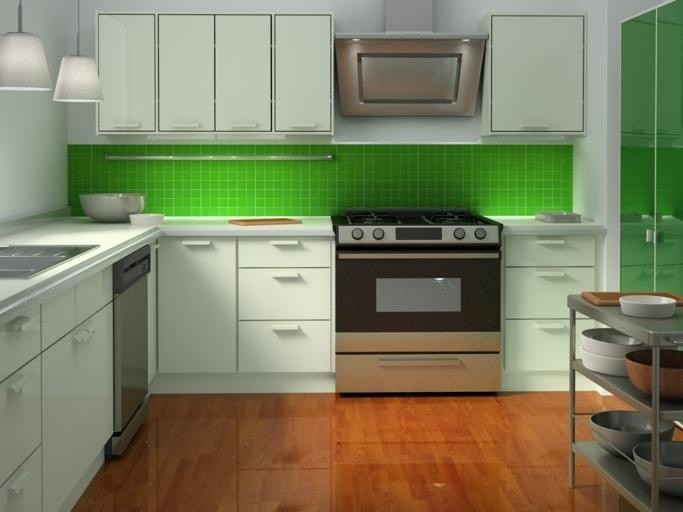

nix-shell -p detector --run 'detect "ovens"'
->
[332,250,502,396]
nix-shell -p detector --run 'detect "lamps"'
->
[52,0,105,103]
[0,0,54,92]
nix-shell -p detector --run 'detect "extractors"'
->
[334,1,488,117]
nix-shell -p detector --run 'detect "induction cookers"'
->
[0,245,100,280]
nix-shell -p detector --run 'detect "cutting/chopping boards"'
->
[580,290,683,307]
[228,218,299,226]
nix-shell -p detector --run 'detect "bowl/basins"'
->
[590,410,675,458]
[77,192,146,223]
[632,443,682,495]
[579,327,677,379]
[625,349,683,401]
[617,294,678,320]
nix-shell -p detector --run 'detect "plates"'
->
[129,213,164,225]
[536,212,582,224]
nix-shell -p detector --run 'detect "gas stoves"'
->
[329,210,503,248]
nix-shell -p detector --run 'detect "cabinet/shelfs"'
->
[621,222,683,299]
[153,228,335,396]
[567,292,683,512]
[95,8,335,136]
[481,12,588,136]
[2,228,156,512]
[500,226,607,393]
